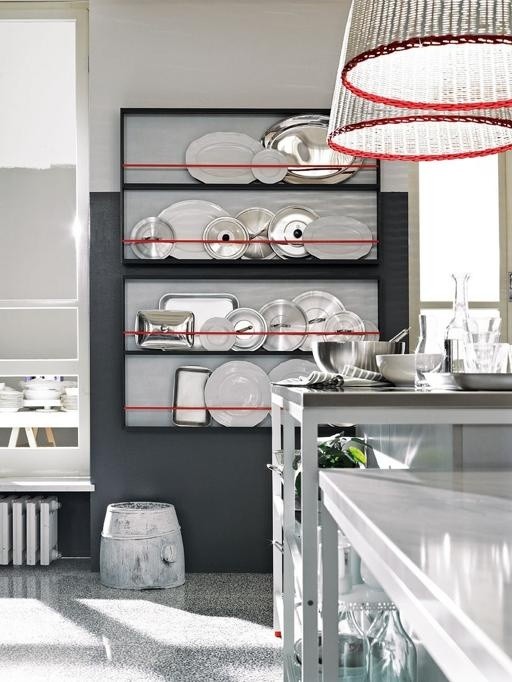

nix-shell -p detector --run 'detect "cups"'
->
[465,343,509,374]
[471,330,500,342]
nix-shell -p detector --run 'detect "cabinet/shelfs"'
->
[120,107,410,434]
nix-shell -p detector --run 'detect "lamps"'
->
[329,0,512,162]
[344,0,511,110]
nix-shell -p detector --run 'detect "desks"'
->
[267,381,511,682]
[0,409,78,448]
[318,468,512,682]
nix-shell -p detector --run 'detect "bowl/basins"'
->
[375,352,443,388]
[312,340,407,387]
[294,632,367,681]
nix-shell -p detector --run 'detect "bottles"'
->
[334,601,419,682]
[445,272,483,374]
[415,313,443,394]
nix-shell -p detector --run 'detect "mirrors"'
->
[2,17,81,303]
[1,306,82,361]
[0,374,80,448]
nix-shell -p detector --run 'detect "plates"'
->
[186,132,264,184]
[200,318,235,351]
[204,359,273,428]
[267,359,323,426]
[362,320,380,341]
[252,150,290,184]
[0,377,79,412]
[302,214,373,260]
[157,200,233,260]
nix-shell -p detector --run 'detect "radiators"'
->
[0,497,63,566]
